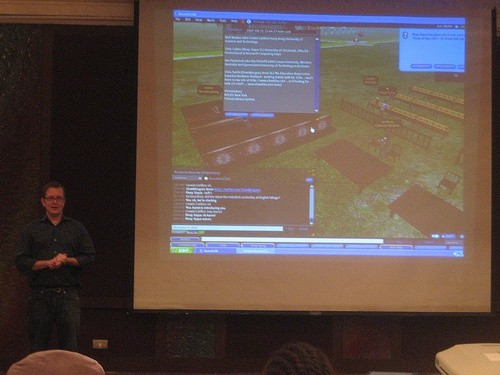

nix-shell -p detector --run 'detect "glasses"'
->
[43,196,64,201]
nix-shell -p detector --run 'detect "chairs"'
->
[385,143,403,163]
[437,170,461,195]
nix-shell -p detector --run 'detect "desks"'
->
[316,140,392,195]
[387,184,463,239]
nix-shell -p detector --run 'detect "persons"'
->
[14,182,96,351]
[265,341,334,375]
